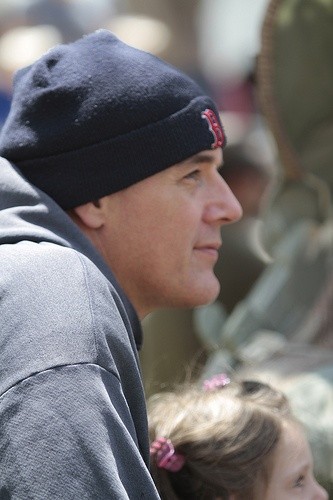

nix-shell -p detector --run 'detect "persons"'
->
[139,111,278,390]
[0,29,249,500]
[139,373,331,500]
[198,219,331,498]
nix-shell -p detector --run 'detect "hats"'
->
[0,27,227,212]
